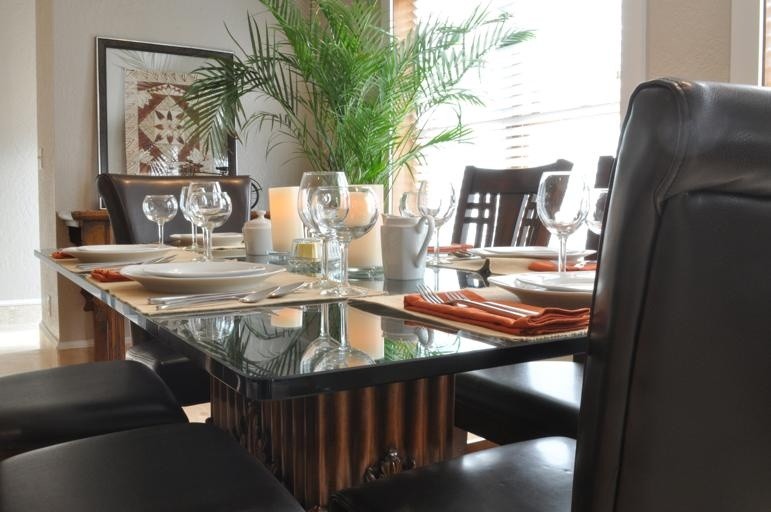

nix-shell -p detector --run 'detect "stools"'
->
[0,354,189,461]
[0,421,310,512]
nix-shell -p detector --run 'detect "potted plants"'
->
[171,0,537,271]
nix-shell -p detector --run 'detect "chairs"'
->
[449,150,619,457]
[327,73,770,512]
[444,155,576,259]
[91,174,257,411]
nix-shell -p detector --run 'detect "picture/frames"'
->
[93,35,237,209]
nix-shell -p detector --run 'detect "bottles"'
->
[241,210,274,257]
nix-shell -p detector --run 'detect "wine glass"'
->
[537,171,590,285]
[295,171,384,298]
[298,298,377,372]
[177,181,232,262]
[417,179,455,267]
[143,194,179,246]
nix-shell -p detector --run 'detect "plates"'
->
[170,232,243,247]
[469,244,596,260]
[64,244,185,263]
[484,272,599,310]
[120,261,285,293]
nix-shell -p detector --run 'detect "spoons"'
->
[148,281,305,305]
[155,284,281,311]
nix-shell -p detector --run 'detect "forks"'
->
[416,284,527,321]
[444,288,539,317]
[77,251,178,268]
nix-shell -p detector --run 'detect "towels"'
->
[425,242,471,257]
[524,258,594,272]
[52,247,71,260]
[404,288,591,340]
[92,265,131,287]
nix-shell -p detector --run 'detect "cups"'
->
[190,315,235,342]
[589,189,609,236]
[381,282,434,349]
[269,185,308,253]
[380,216,436,281]
[399,181,422,219]
[290,237,324,280]
